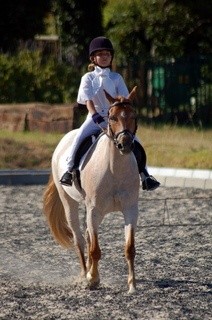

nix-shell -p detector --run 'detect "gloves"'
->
[92,112,105,125]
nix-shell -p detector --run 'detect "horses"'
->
[42,85,140,295]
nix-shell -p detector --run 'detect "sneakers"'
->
[141,175,160,191]
[59,171,73,187]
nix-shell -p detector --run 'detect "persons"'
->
[59,37,160,190]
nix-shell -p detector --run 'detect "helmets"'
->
[89,36,115,56]
